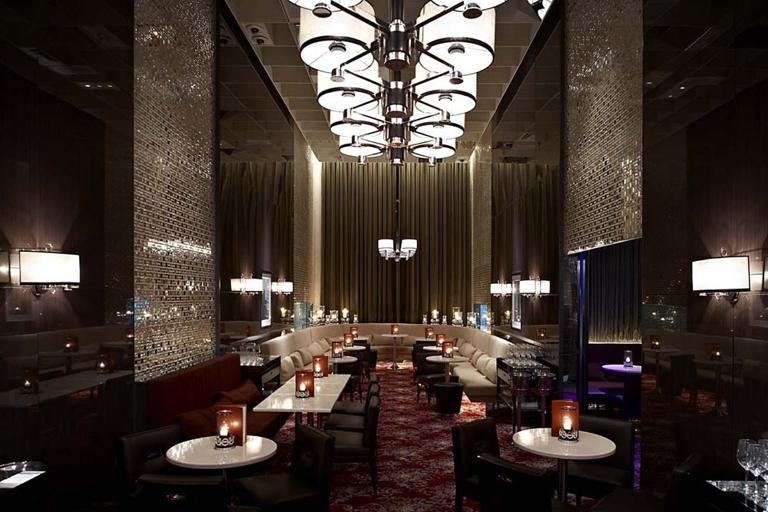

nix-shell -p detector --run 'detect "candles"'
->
[307,305,359,324]
[422,307,476,328]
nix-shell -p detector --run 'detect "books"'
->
[350,326,357,338]
[313,356,328,376]
[436,334,446,346]
[551,400,581,437]
[332,342,343,358]
[296,370,314,397]
[391,325,400,334]
[442,343,452,358]
[344,333,354,347]
[425,327,433,338]
[215,403,247,447]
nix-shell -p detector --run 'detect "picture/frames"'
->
[260,272,272,329]
[510,272,522,331]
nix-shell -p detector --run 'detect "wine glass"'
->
[737,438,768,503]
[503,342,538,368]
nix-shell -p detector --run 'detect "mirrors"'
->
[490,0,565,332]
[0,1,137,511]
[214,8,296,357]
[642,1,767,488]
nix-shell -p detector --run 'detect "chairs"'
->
[115,319,767,512]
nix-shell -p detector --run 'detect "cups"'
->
[238,342,261,364]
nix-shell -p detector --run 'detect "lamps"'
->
[7,247,82,296]
[689,253,750,306]
[283,1,508,169]
[537,281,550,298]
[242,278,264,296]
[377,238,418,262]
[279,281,293,295]
[271,281,279,295]
[490,283,502,298]
[502,283,513,297]
[230,278,243,292]
[518,280,536,297]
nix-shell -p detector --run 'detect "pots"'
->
[505,372,531,392]
[535,372,560,392]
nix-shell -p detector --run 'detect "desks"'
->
[0,323,133,460]
